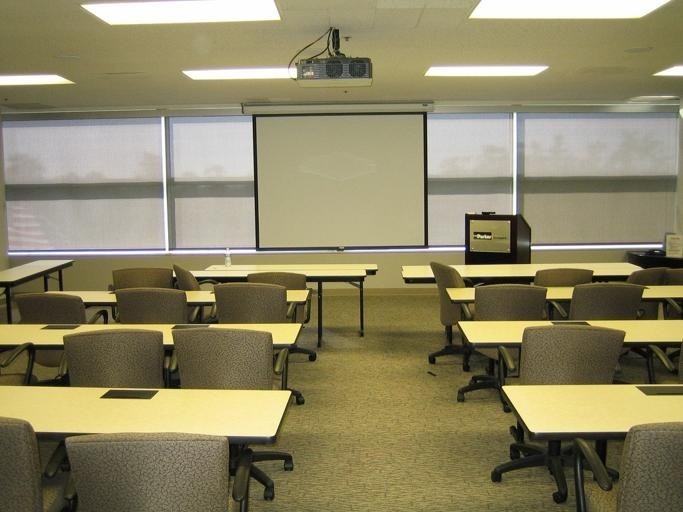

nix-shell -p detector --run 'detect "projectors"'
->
[296,58,373,87]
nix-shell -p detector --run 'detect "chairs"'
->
[0,264,313,511]
[400,263,683,511]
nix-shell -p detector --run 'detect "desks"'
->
[0,259,77,324]
[173,263,378,349]
